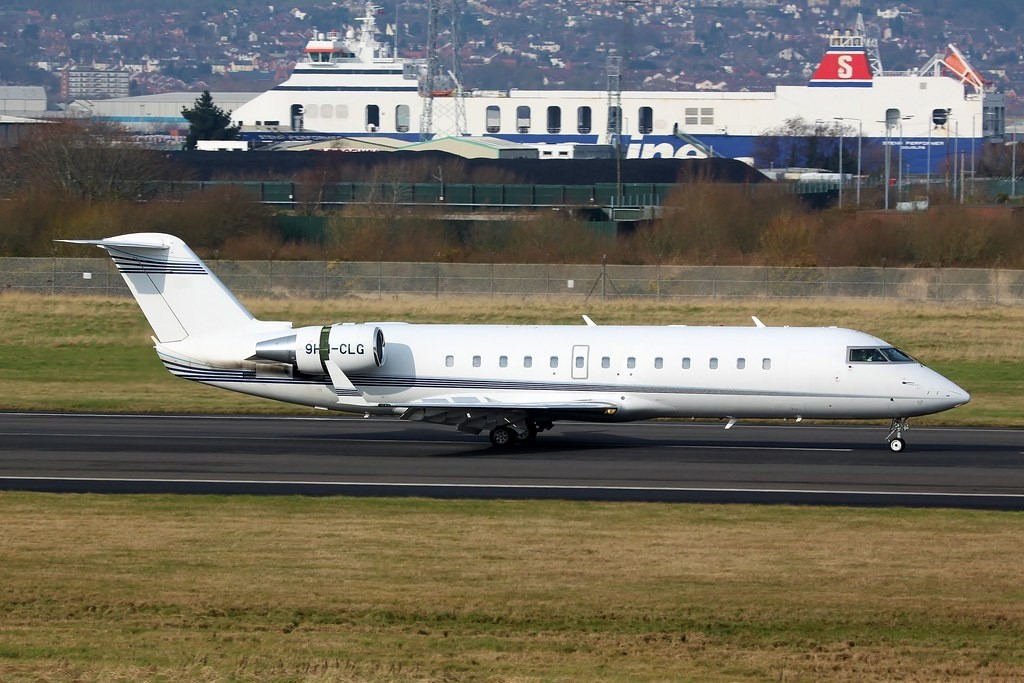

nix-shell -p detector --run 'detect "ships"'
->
[226,2,989,187]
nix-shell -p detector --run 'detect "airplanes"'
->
[52,229,972,453]
